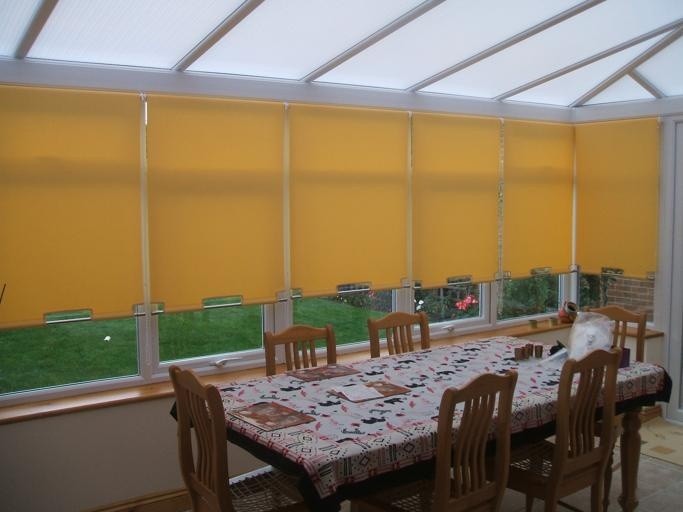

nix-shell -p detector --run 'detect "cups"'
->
[528,319,537,328]
[548,317,558,326]
[514,342,545,360]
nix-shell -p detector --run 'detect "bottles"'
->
[558,299,577,324]
